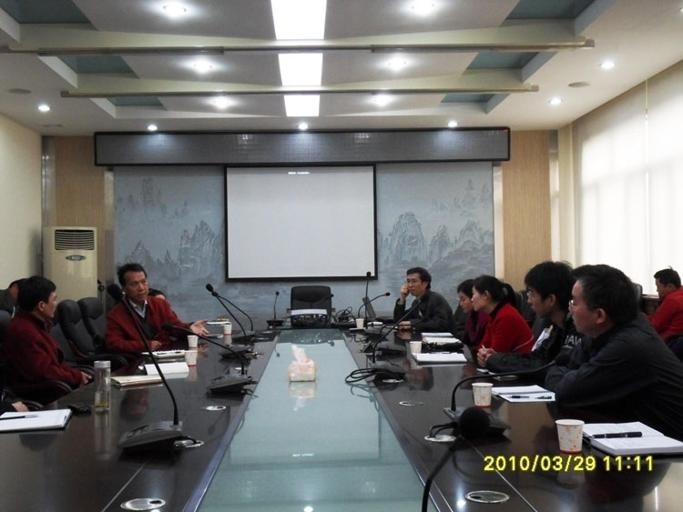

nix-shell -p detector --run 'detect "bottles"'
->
[93,361,111,409]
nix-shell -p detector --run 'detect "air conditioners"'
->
[41,226,97,301]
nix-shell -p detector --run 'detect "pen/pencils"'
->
[592,431,642,438]
[157,351,181,355]
[507,395,552,399]
[0,415,26,421]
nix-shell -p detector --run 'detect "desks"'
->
[0,312,683,511]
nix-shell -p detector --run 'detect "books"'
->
[138,362,189,376]
[410,352,468,366]
[581,421,683,458]
[110,375,166,387]
[153,350,185,359]
[0,409,73,434]
[492,384,556,403]
[421,332,462,345]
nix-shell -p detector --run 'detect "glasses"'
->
[526,290,535,299]
[568,299,576,309]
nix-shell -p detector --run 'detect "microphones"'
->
[274,291,280,320]
[160,321,246,375]
[450,351,570,411]
[357,292,391,318]
[365,272,371,328]
[205,282,254,331]
[105,282,179,425]
[372,293,429,363]
[325,293,334,326]
[421,404,491,512]
[211,292,248,346]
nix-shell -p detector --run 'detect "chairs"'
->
[52,324,78,367]
[78,296,109,342]
[56,299,98,353]
[290,285,332,329]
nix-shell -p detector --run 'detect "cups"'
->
[186,352,197,363]
[223,325,230,333]
[409,340,421,354]
[356,318,362,328]
[474,382,492,407]
[188,335,197,346]
[555,418,584,452]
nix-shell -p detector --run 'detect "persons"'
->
[545,264,683,442]
[1,275,92,385]
[0,279,26,320]
[147,288,178,319]
[393,267,454,330]
[471,275,535,353]
[105,263,209,353]
[457,279,490,351]
[0,389,29,419]
[477,261,585,374]
[646,269,683,342]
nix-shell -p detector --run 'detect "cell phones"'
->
[67,400,91,415]
